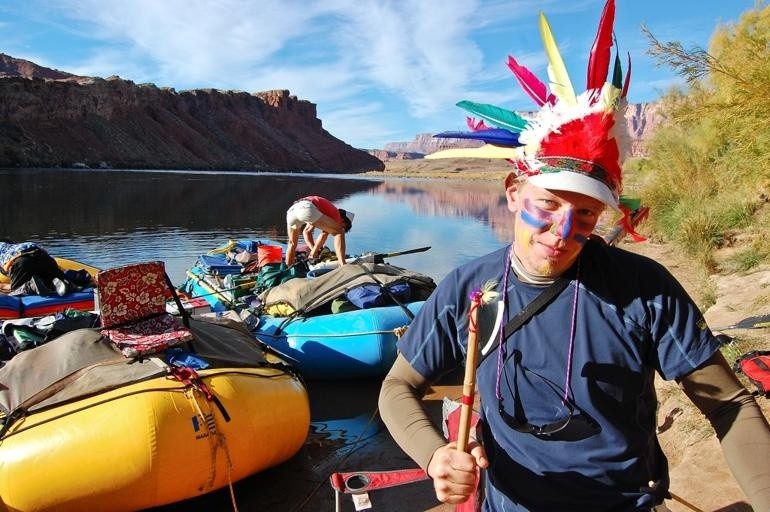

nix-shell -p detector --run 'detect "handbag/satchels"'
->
[734,349,770,397]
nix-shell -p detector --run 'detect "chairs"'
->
[329,393,484,512]
[96,260,195,359]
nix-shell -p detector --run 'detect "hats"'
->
[344,208,357,228]
[516,147,627,214]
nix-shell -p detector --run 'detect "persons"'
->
[0,239,76,297]
[284,195,352,267]
[376,169,769,511]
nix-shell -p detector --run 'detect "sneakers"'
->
[50,276,72,298]
[28,274,50,297]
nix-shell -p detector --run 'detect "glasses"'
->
[494,345,578,438]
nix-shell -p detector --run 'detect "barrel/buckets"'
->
[257,245,282,267]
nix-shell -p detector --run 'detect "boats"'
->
[2,228,315,506]
[181,235,441,387]
